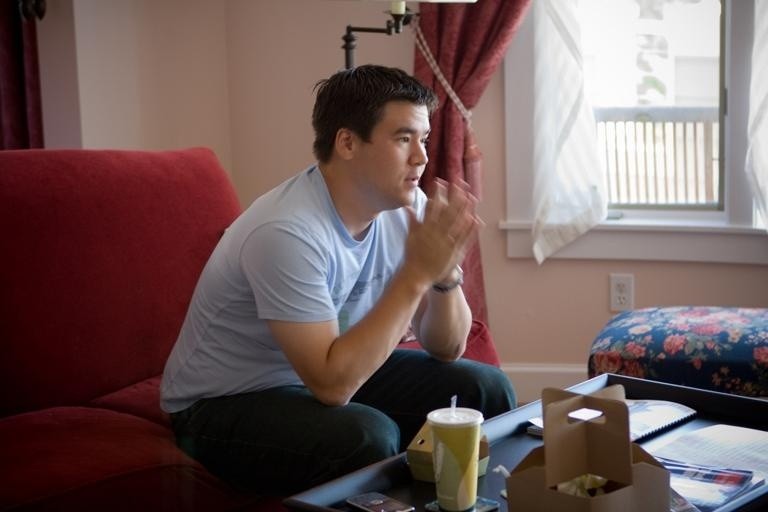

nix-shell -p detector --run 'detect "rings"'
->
[444,232,458,248]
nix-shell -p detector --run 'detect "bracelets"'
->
[431,264,466,293]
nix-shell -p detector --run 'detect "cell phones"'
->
[346,491,415,512]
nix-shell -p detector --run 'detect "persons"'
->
[160,64,518,502]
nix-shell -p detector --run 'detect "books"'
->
[524,394,696,450]
[650,455,754,512]
[734,475,765,500]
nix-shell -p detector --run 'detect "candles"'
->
[391,1,406,13]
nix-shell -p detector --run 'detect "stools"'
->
[588,306,768,400]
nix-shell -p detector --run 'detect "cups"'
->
[428,407,482,510]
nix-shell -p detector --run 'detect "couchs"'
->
[0,146,501,512]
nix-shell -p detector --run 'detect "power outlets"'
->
[608,273,636,312]
